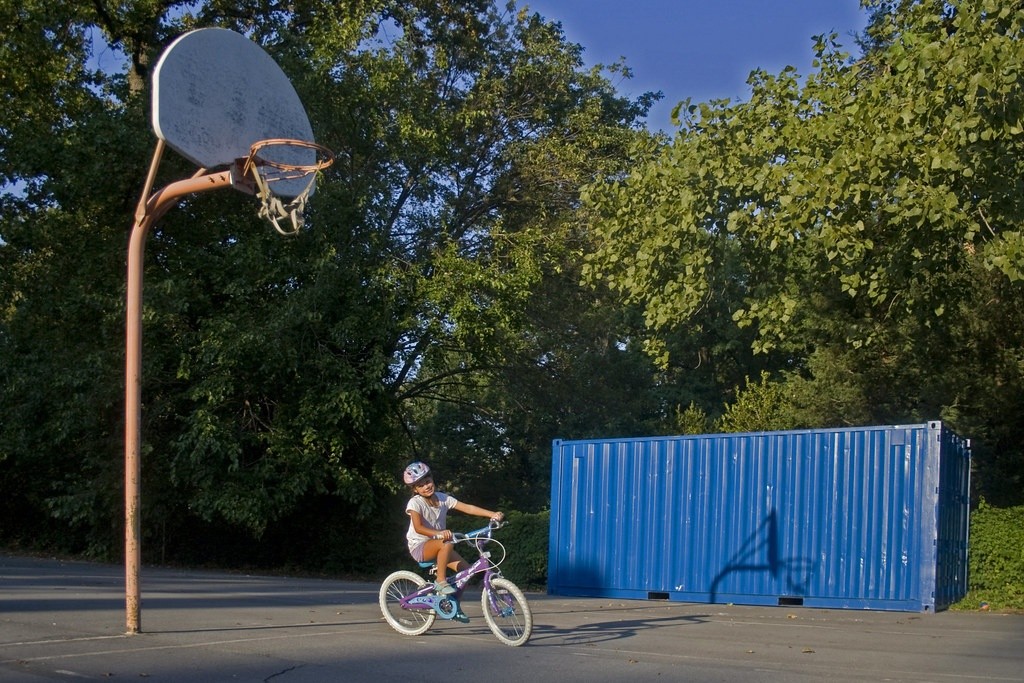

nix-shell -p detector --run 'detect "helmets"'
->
[404,461,431,485]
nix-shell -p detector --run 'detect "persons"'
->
[404,462,505,623]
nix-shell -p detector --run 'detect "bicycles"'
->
[379,517,532,646]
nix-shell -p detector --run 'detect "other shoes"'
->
[449,608,470,623]
[434,580,456,594]
